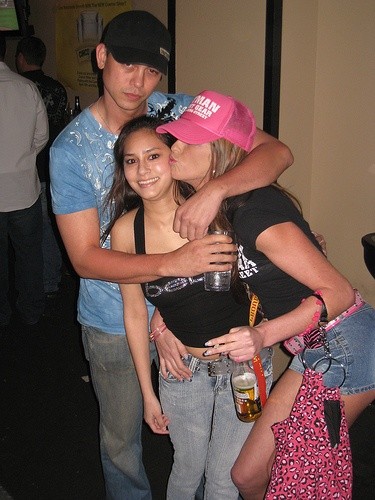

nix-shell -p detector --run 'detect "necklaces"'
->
[96,96,113,134]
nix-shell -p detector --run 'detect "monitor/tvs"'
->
[0,0,28,37]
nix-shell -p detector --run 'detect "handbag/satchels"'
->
[263,292,352,500]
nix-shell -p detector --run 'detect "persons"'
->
[48,8,295,500]
[15,36,69,296]
[0,32,52,329]
[98,114,274,500]
[153,86,375,500]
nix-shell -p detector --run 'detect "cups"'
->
[204,230,235,292]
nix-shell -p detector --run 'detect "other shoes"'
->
[21,299,44,326]
[45,292,57,297]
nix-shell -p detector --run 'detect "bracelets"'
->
[149,322,166,342]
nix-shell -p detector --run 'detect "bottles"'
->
[73,96,82,117]
[230,359,262,423]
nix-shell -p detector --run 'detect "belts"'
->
[281,289,366,355]
[181,357,240,376]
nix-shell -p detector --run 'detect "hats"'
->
[100,10,171,76]
[156,90,256,152]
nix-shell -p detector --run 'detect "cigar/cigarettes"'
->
[212,342,220,350]
